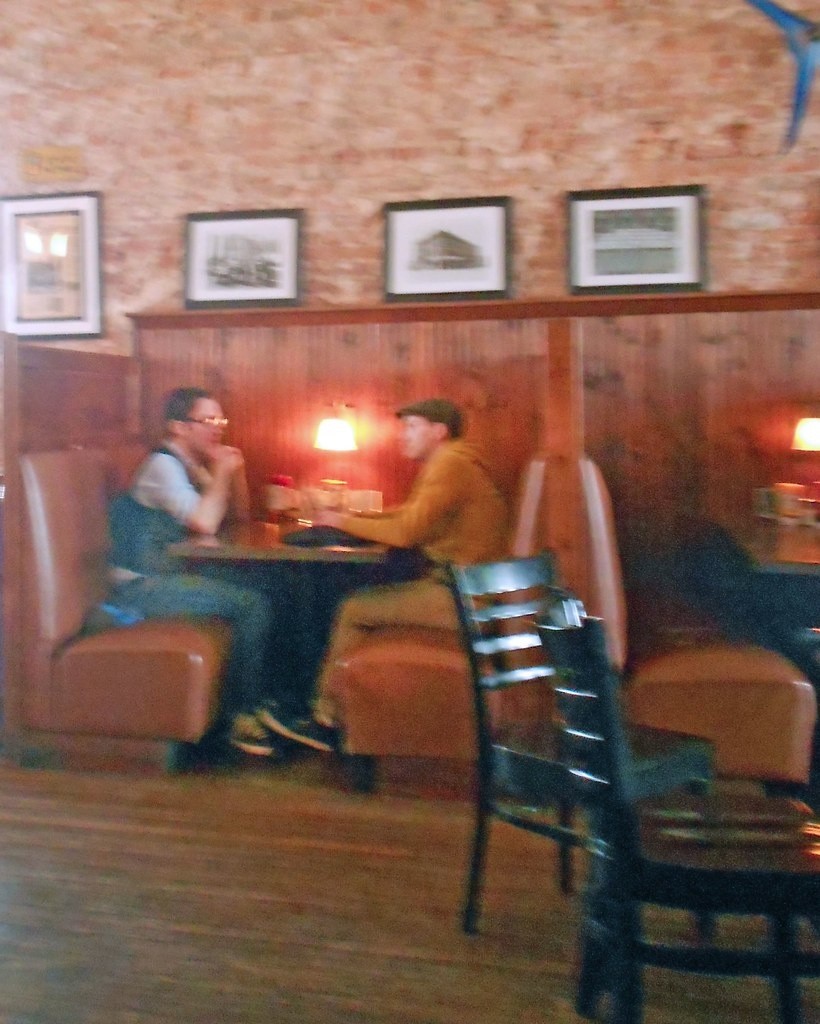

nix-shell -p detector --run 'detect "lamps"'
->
[314,420,358,491]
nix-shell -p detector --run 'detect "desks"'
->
[709,500,820,636]
[170,516,391,776]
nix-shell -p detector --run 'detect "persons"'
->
[258,399,509,751]
[104,383,280,756]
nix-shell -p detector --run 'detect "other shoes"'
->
[228,731,285,757]
[256,703,335,753]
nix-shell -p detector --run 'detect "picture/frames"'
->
[185,208,303,310]
[568,184,708,295]
[0,191,104,341]
[384,196,513,303]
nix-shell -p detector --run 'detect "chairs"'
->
[523,617,820,1024]
[448,547,718,935]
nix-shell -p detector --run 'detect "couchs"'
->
[1,442,820,801]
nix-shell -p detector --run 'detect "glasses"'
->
[173,414,230,428]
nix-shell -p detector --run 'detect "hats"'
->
[395,398,465,436]
